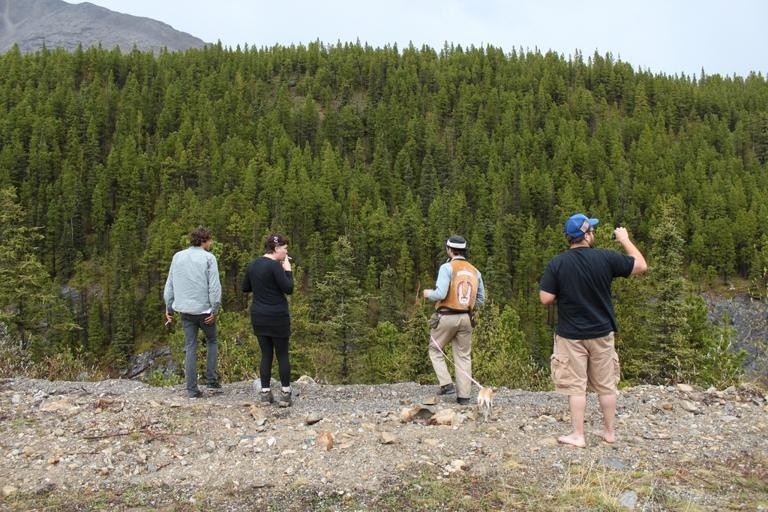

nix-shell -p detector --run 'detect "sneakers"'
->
[438,383,469,404]
[279,391,291,407]
[189,383,222,399]
[261,391,273,406]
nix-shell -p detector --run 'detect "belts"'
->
[439,309,468,315]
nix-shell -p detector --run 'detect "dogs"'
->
[477,386,498,423]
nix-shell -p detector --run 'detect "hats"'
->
[564,214,599,239]
[447,237,466,249]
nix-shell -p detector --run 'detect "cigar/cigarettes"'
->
[446,258,450,263]
[288,257,292,260]
[165,319,171,326]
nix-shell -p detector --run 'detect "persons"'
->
[241,234,294,408]
[163,225,223,397]
[423,236,486,405]
[539,212,649,448]
